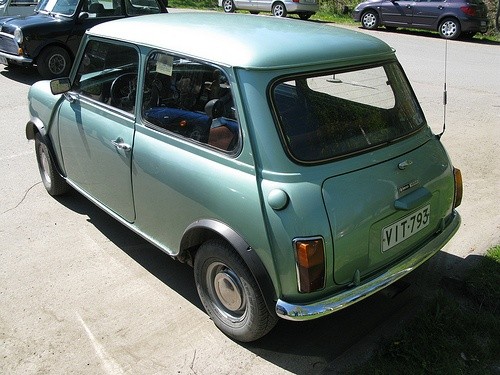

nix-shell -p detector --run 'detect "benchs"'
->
[288,108,383,151]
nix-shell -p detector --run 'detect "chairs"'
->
[88,3,104,17]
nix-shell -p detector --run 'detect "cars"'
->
[351,0,490,41]
[0,0,40,25]
[217,0,320,21]
[25,11,464,344]
[0,0,169,81]
